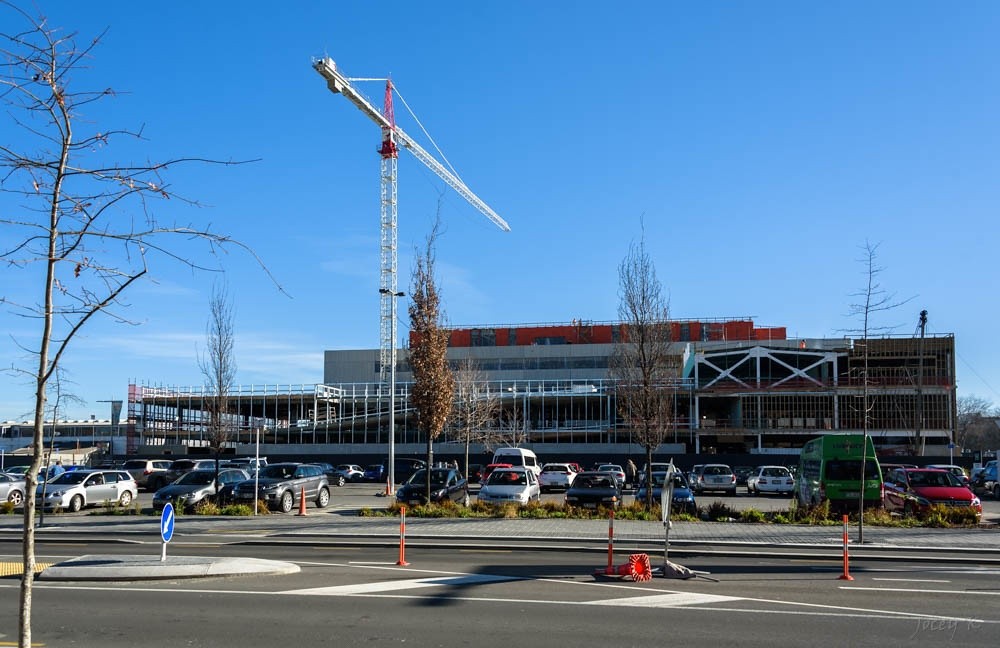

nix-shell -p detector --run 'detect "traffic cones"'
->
[295,486,312,516]
[590,552,652,581]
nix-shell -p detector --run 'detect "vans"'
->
[799,435,885,514]
[492,448,543,478]
[380,459,429,485]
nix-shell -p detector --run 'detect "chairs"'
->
[599,479,610,487]
[674,479,680,488]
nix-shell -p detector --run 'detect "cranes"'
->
[311,57,512,391]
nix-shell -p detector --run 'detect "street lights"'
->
[378,288,408,499]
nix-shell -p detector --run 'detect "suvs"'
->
[145,458,218,492]
[123,458,176,491]
[232,462,331,512]
[91,460,127,471]
[35,469,139,513]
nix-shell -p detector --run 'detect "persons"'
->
[800,339,806,348]
[625,459,636,489]
[51,461,65,478]
[452,460,458,470]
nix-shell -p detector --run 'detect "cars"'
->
[537,463,579,493]
[972,460,1000,500]
[335,464,365,479]
[476,466,543,508]
[5,465,47,479]
[686,464,705,493]
[219,457,268,477]
[631,471,698,517]
[593,462,614,473]
[308,462,348,486]
[0,472,28,508]
[564,472,624,512]
[733,466,754,486]
[396,468,472,507]
[152,467,255,507]
[596,465,627,489]
[38,465,90,483]
[362,465,386,481]
[746,466,796,497]
[635,462,683,487]
[694,464,737,497]
[480,463,520,485]
[881,469,982,523]
[878,462,971,492]
